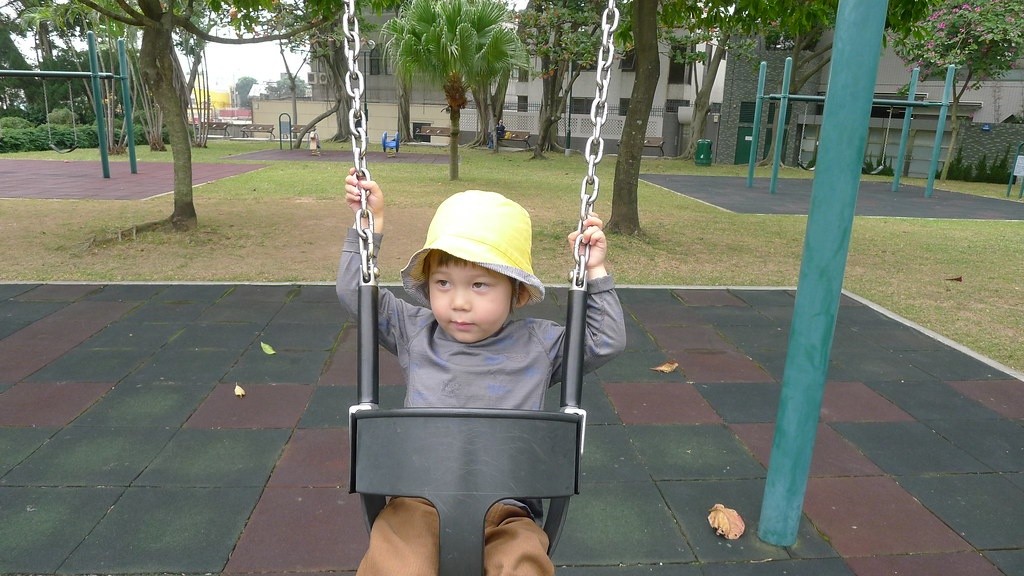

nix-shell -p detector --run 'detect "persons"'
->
[488,120,506,149]
[336,167,628,576]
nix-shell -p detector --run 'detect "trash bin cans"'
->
[410,121,434,143]
[694,139,713,166]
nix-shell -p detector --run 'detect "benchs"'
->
[282,125,316,139]
[500,131,530,148]
[195,122,230,136]
[240,124,275,139]
[415,126,450,143]
[643,137,664,157]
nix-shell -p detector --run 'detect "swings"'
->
[798,100,825,172]
[861,107,893,175]
[41,79,80,154]
[335,0,625,576]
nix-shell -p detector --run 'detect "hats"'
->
[400,190,545,311]
